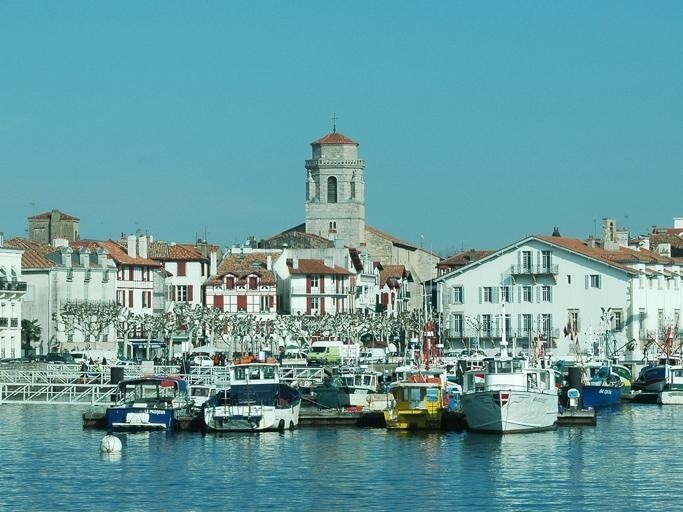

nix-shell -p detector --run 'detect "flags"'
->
[562,319,571,337]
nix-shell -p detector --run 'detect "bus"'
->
[44,353,85,363]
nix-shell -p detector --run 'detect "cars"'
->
[188,356,214,366]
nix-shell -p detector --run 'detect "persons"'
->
[79,351,228,388]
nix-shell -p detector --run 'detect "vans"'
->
[307,340,342,365]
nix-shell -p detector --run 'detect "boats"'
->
[561,358,631,409]
[633,363,683,404]
[204,362,302,430]
[107,377,190,430]
[331,356,558,435]
